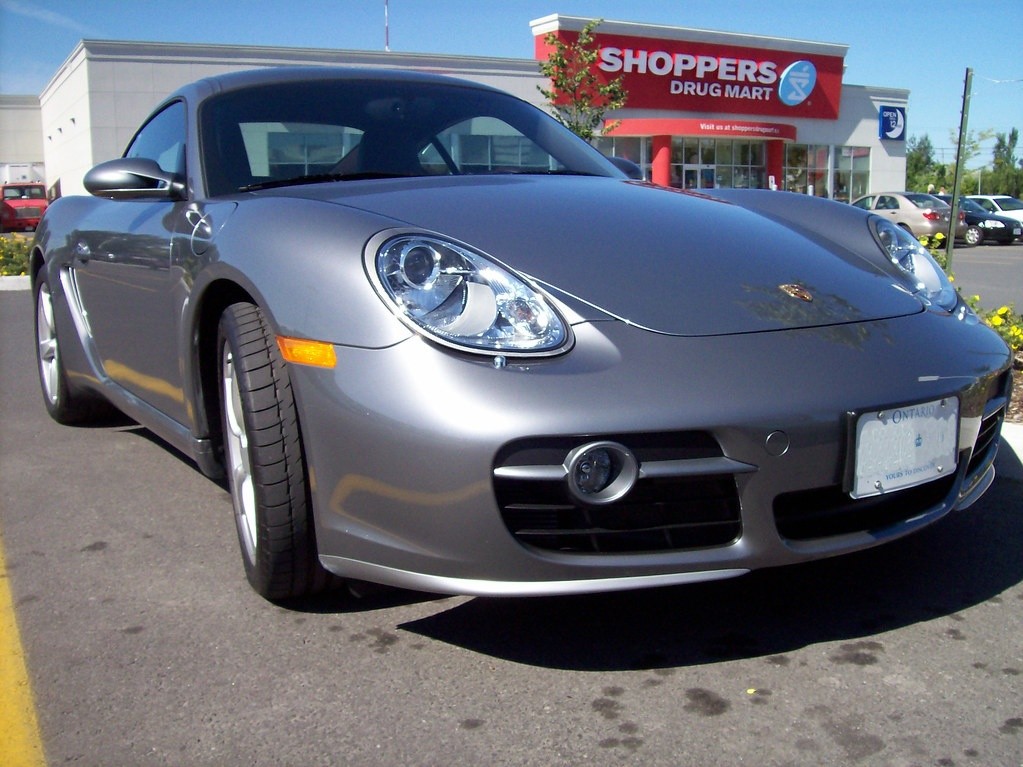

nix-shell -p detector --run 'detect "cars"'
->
[847,191,968,243]
[958,194,1023,229]
[886,193,1023,247]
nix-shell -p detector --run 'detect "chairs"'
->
[203,120,253,198]
[358,127,429,176]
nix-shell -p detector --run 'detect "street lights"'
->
[976,158,1004,196]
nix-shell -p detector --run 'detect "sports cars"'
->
[27,67,1015,610]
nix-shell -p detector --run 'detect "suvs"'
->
[0,181,49,231]
